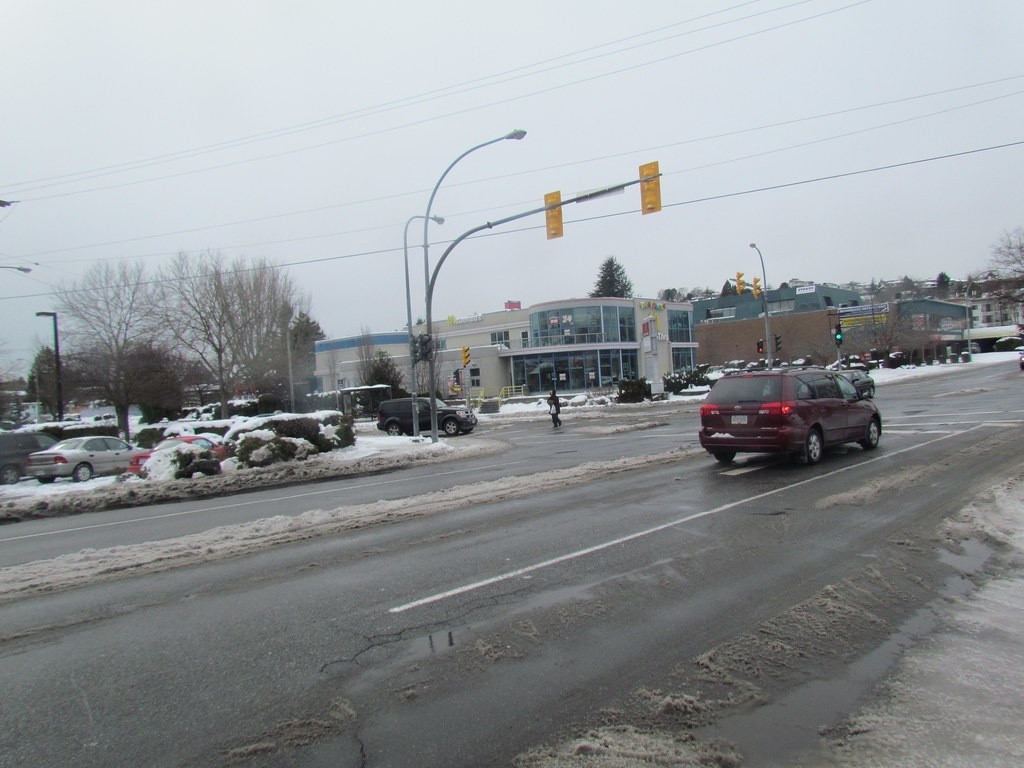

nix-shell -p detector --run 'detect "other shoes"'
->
[559,421,561,426]
[553,426,558,427]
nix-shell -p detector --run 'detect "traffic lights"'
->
[418,335,432,361]
[757,341,764,353]
[736,271,745,294]
[454,370,460,385]
[753,278,762,298]
[463,345,471,366]
[774,334,782,353]
[835,327,842,346]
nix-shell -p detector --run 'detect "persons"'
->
[802,355,813,370]
[547,390,562,427]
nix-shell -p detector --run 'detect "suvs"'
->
[377,397,478,436]
[0,431,60,486]
[698,365,882,467]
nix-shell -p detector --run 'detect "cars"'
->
[966,343,981,353]
[1019,352,1024,370]
[128,434,226,474]
[24,435,151,484]
[836,369,875,399]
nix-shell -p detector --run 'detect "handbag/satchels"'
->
[550,404,556,414]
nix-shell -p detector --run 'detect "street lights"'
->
[35,311,63,421]
[749,242,772,371]
[404,214,446,438]
[965,269,998,362]
[423,129,528,442]
